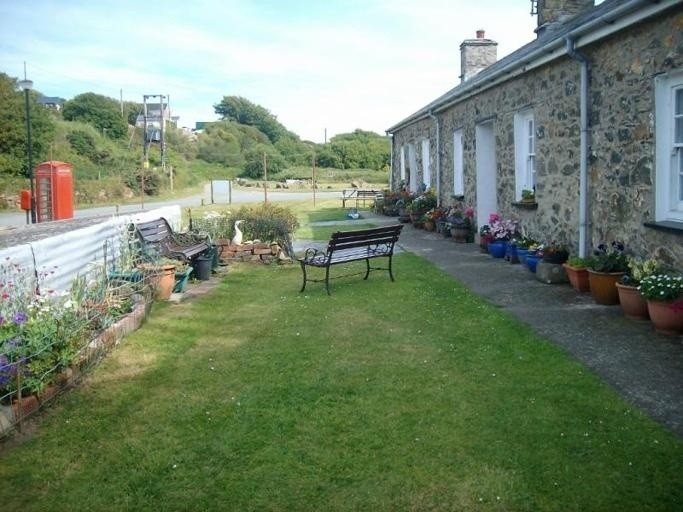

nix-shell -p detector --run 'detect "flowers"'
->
[396,186,683,303]
[0,254,61,392]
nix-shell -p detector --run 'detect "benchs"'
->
[342,189,380,212]
[129,217,212,264]
[297,223,406,299]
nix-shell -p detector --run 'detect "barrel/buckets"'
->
[194,256,213,280]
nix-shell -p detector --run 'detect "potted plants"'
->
[105,219,192,302]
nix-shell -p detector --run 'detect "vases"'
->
[584,270,682,336]
[192,254,213,281]
